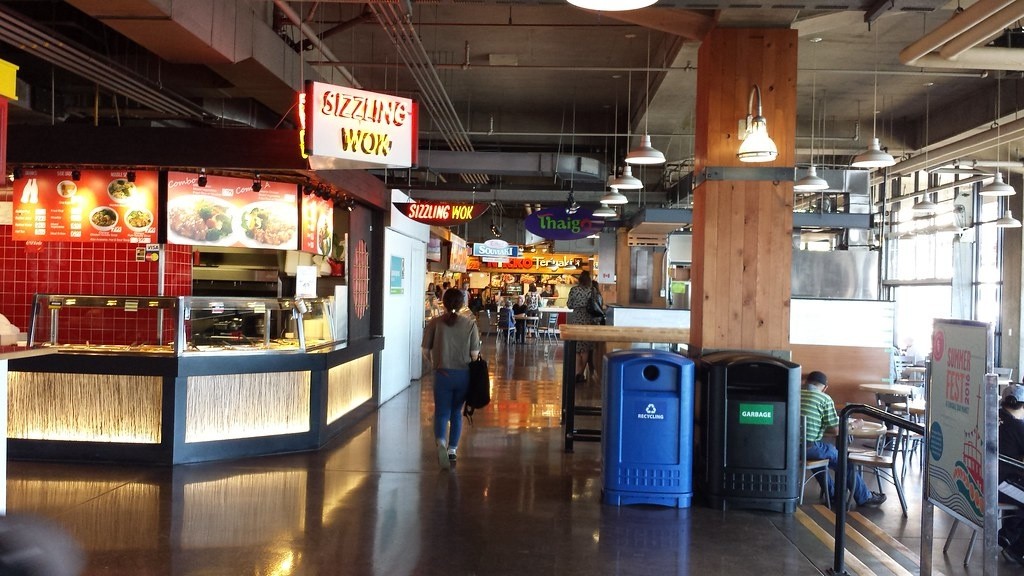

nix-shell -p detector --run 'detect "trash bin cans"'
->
[601,348,696,508]
[700,353,799,513]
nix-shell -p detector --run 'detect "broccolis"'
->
[117,180,125,185]
[197,207,233,241]
[242,207,269,239]
[102,209,116,221]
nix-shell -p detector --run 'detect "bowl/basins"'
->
[124,208,153,232]
[89,206,118,231]
[539,299,547,307]
[108,179,136,204]
[17,341,27,346]
[285,332,294,338]
[508,287,515,291]
[517,286,521,290]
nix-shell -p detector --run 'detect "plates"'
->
[231,201,298,250]
[57,180,77,198]
[167,194,239,246]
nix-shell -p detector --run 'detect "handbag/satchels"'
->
[586,287,604,317]
[463,351,491,425]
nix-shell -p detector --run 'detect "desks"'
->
[891,401,926,415]
[822,416,883,438]
[904,366,926,373]
[997,378,1013,385]
[859,382,916,395]
[515,316,539,345]
[558,323,691,452]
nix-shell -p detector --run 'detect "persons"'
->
[799,372,887,505]
[459,281,470,307]
[587,279,603,375]
[540,284,554,306]
[567,269,599,384]
[421,288,481,470]
[428,282,458,301]
[482,286,492,309]
[498,301,516,343]
[525,283,542,338]
[511,295,529,343]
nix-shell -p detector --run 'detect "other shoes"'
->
[438,441,457,468]
[576,374,584,383]
[860,491,887,506]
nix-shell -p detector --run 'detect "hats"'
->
[807,371,827,385]
[1000,384,1024,402]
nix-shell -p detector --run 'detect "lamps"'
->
[519,245,549,252]
[792,17,1023,227]
[303,180,357,212]
[736,83,778,163]
[563,30,667,216]
[197,172,207,187]
[71,168,80,181]
[253,178,261,192]
[128,168,136,182]
[13,166,22,180]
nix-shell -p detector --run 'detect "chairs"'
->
[945,504,1020,566]
[996,368,1012,399]
[893,354,924,386]
[486,309,560,347]
[798,387,924,519]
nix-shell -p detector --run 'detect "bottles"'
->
[21,179,38,204]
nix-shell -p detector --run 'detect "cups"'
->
[295,299,307,313]
